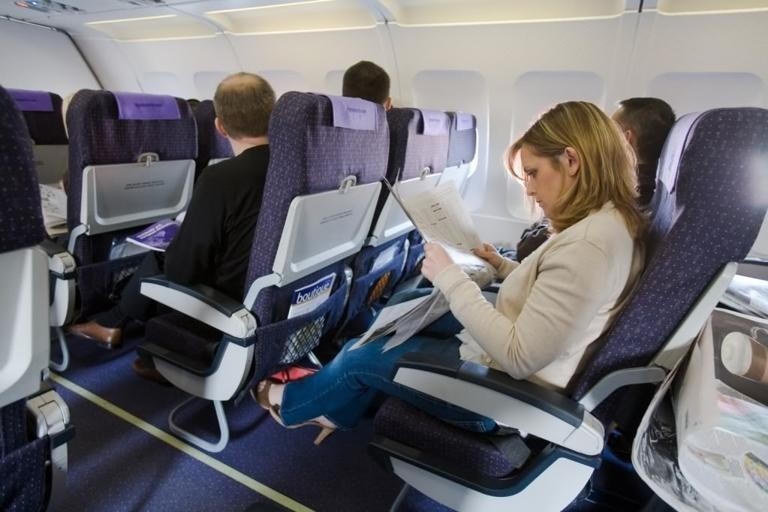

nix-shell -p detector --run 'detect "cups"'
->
[722,326,768,385]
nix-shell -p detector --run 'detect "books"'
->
[281,272,335,363]
[126,211,187,256]
[36,182,68,235]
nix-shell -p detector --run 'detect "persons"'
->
[340,59,392,111]
[249,102,647,448]
[484,96,679,260]
[70,70,276,388]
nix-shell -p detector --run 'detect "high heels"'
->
[249,380,338,445]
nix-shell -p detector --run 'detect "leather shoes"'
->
[132,359,174,387]
[65,319,122,350]
